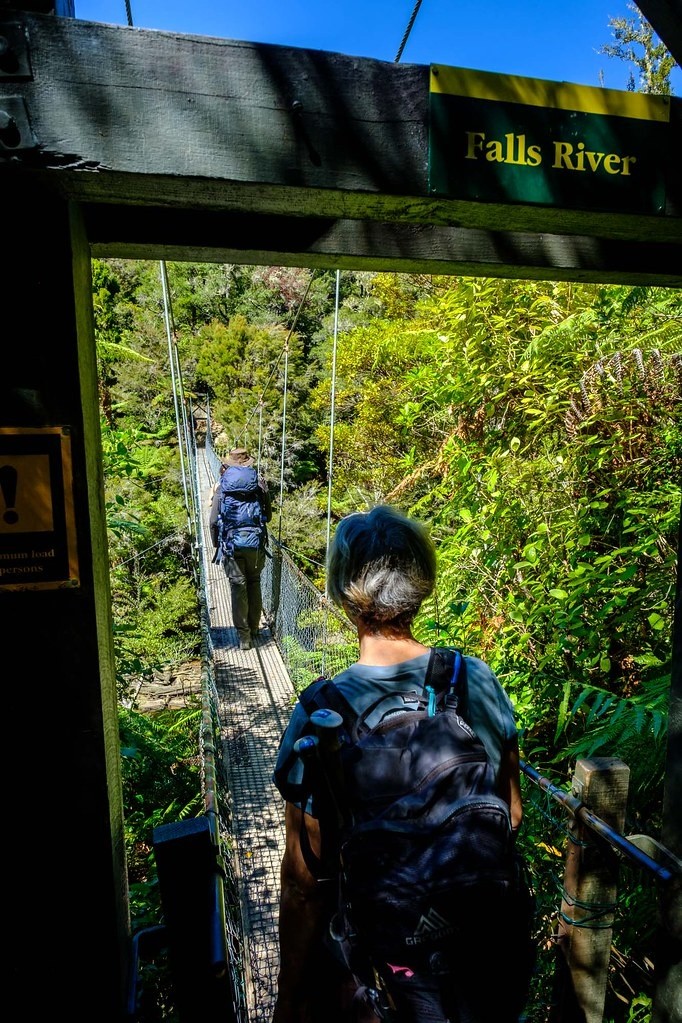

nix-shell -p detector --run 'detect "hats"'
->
[224,448,254,468]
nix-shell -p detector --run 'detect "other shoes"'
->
[240,640,252,650]
[250,634,265,647]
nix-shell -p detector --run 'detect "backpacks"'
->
[300,644,533,989]
[211,465,272,564]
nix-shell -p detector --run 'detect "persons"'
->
[209,447,272,650]
[272,505,523,1023]
